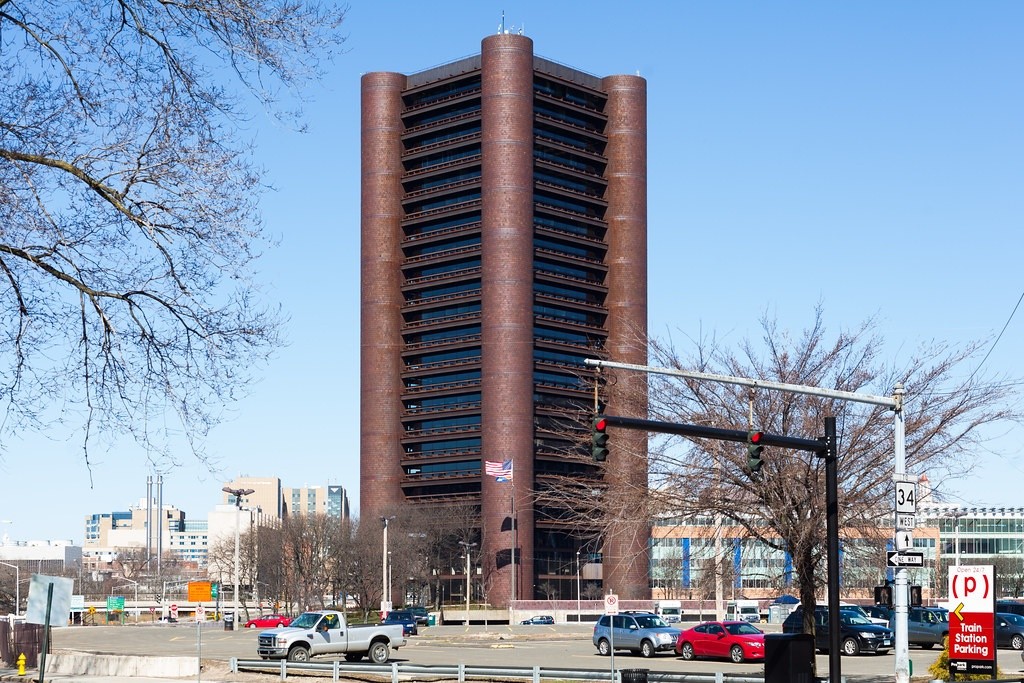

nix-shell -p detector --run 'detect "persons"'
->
[320,619,327,626]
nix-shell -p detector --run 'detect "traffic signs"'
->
[885,551,925,569]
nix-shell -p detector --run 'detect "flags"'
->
[485,459,511,479]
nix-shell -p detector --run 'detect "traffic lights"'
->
[592,417,610,461]
[747,429,765,471]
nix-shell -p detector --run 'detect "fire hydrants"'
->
[16,652,27,676]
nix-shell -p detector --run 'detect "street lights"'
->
[459,541,478,627]
[576,551,581,623]
[379,515,396,621]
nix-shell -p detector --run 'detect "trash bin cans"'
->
[224,612,234,631]
[618,668,650,683]
[762,631,817,683]
[429,615,435,624]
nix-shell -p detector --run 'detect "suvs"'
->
[781,599,895,657]
[592,609,682,658]
[520,615,555,625]
[889,605,949,649]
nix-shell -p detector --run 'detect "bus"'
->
[724,599,761,623]
[654,600,684,623]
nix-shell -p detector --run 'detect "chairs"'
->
[334,619,340,628]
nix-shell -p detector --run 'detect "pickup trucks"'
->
[256,608,407,664]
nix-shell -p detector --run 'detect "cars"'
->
[995,611,1024,650]
[243,613,294,628]
[995,599,1024,617]
[674,619,768,663]
[405,606,428,626]
[381,609,419,636]
